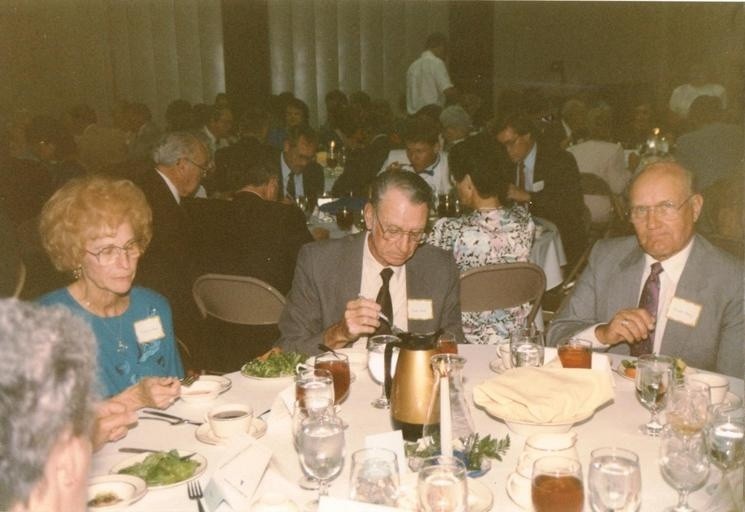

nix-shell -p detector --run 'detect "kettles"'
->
[383,330,443,439]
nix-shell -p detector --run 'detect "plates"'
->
[398,473,494,512]
[505,473,603,511]
[489,359,507,374]
[86,347,296,512]
[504,418,574,445]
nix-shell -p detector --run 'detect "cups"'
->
[437,191,455,219]
[435,331,459,361]
[585,446,641,512]
[297,370,334,419]
[554,338,592,369]
[493,339,512,368]
[510,328,544,367]
[420,453,466,512]
[529,456,586,512]
[348,449,399,506]
[516,434,580,478]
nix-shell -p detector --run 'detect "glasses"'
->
[79,240,140,266]
[375,212,424,243]
[629,199,688,219]
[188,158,209,178]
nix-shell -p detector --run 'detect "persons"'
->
[0,291,109,511]
[668,52,731,115]
[24,169,189,412]
[404,32,466,119]
[2,90,744,378]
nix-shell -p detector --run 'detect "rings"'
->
[169,398,176,403]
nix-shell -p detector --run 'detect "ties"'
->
[366,268,393,347]
[631,262,664,357]
[286,172,296,200]
[518,163,525,192]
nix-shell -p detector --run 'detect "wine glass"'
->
[327,148,348,176]
[301,415,345,510]
[293,189,365,237]
[365,336,400,408]
[313,351,351,432]
[658,372,745,512]
[296,395,337,491]
[635,355,674,436]
